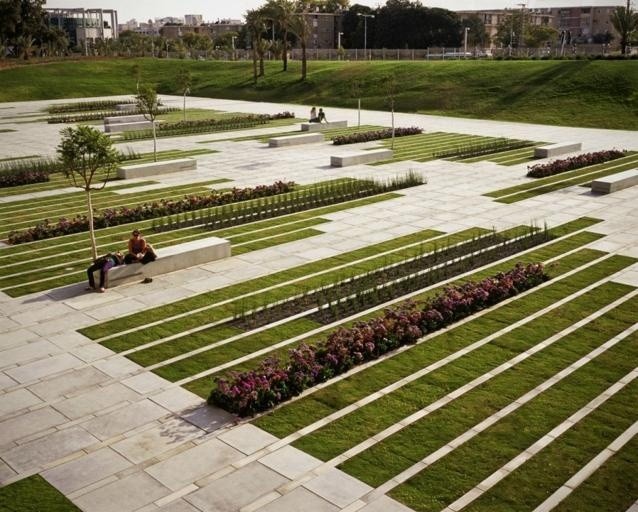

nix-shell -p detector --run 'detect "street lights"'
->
[357,12,375,47]
[464,27,471,52]
[232,36,237,59]
[337,31,344,49]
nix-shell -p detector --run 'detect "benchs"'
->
[534,140,582,159]
[330,148,394,168]
[85,236,232,289]
[115,104,137,111]
[103,114,160,134]
[591,169,638,193]
[268,122,347,148]
[116,157,198,181]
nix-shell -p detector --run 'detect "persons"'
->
[310,106,316,119]
[84,250,125,293]
[123,230,157,265]
[308,109,330,126]
